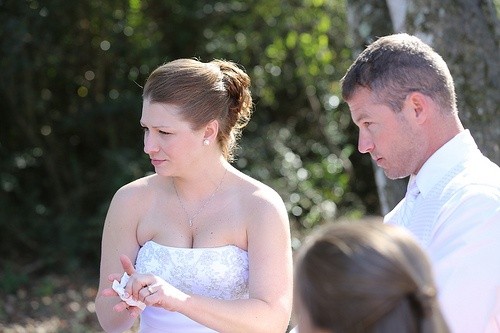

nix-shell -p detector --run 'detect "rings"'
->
[146,285,154,294]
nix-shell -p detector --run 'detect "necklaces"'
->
[169,161,230,228]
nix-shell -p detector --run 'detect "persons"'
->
[87,53,294,333]
[293,214,452,332]
[338,32,500,331]
